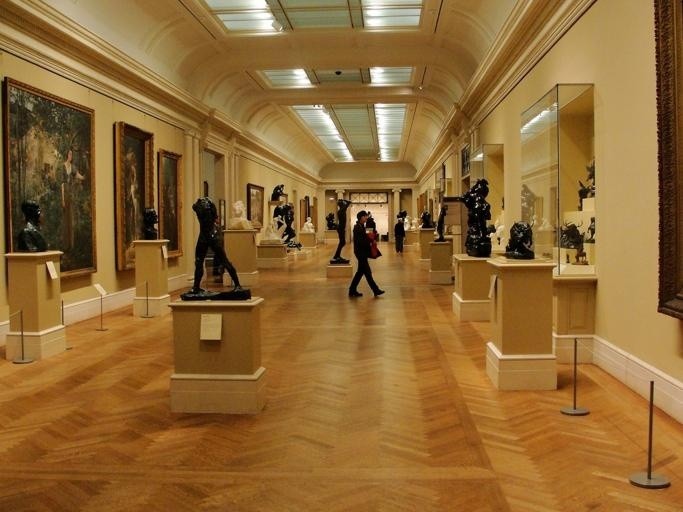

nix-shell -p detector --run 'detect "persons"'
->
[187,196,242,293]
[141,206,159,241]
[331,198,351,261]
[124,141,140,226]
[367,211,371,218]
[302,216,316,233]
[13,199,49,253]
[227,199,261,231]
[57,148,89,253]
[270,182,301,251]
[348,210,384,297]
[393,217,406,256]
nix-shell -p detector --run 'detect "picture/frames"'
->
[2,74,184,278]
[245,183,264,228]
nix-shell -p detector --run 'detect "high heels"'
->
[349,290,362,296]
[374,289,384,296]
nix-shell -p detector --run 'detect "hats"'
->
[357,211,370,219]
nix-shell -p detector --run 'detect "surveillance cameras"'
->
[335,71,341,75]
